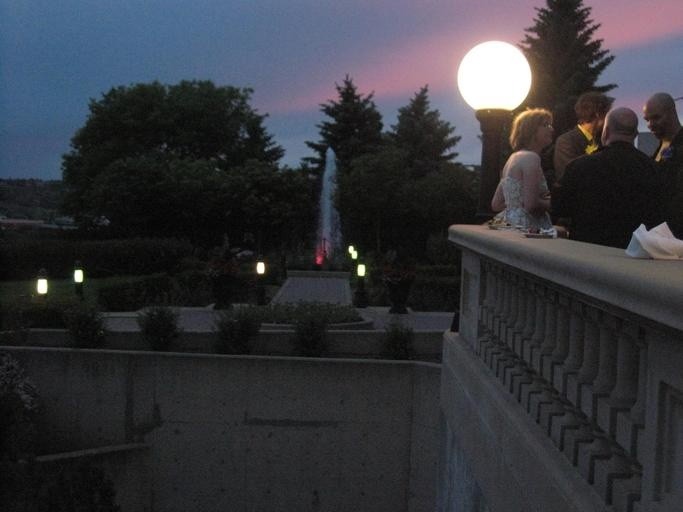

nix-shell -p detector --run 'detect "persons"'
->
[492,107,554,233]
[552,90,611,182]
[552,108,669,249]
[643,92,683,240]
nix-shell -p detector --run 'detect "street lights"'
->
[253,257,268,307]
[33,267,49,299]
[71,263,86,298]
[444,33,536,335]
[344,243,370,309]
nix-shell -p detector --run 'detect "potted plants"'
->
[382,270,416,314]
[281,298,373,355]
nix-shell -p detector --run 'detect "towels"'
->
[624,221,683,260]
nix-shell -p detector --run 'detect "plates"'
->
[489,222,554,238]
